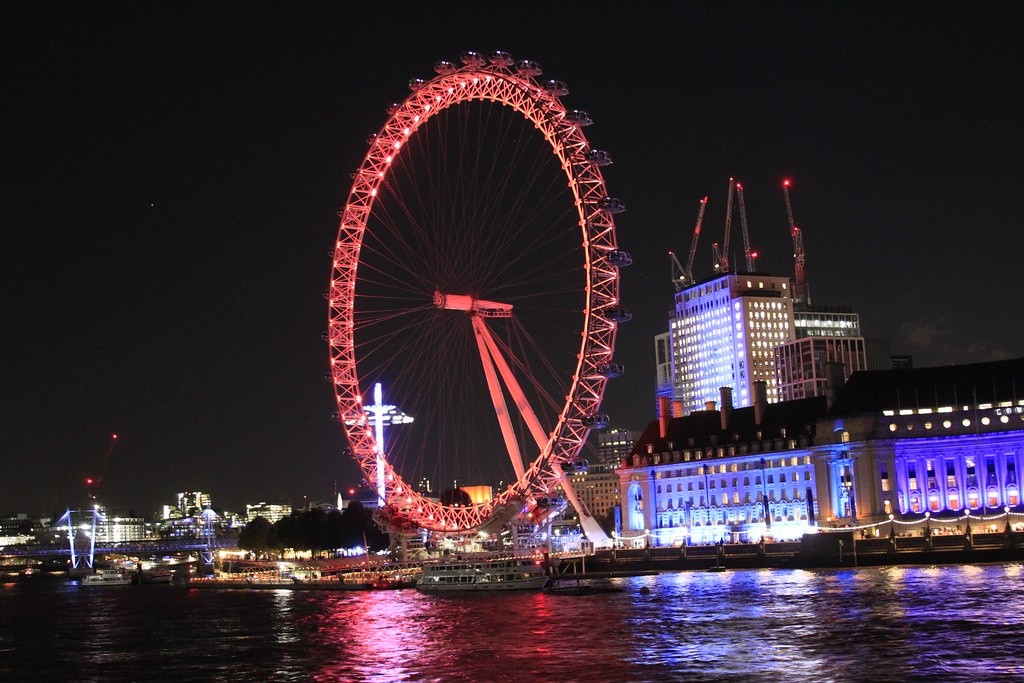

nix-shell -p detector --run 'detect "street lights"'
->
[651,469,660,546]
[703,463,714,542]
[761,457,772,533]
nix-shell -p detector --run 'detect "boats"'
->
[415,562,549,593]
[145,563,174,582]
[82,570,131,585]
